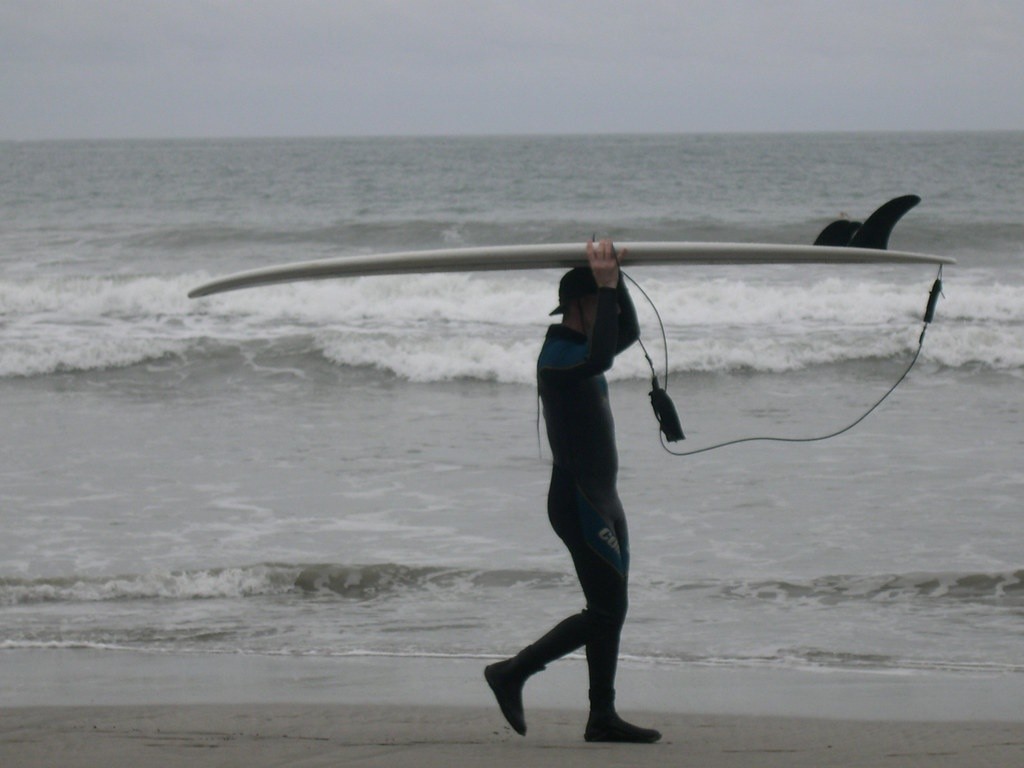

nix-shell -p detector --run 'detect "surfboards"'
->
[183,193,960,300]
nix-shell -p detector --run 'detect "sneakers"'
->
[485,645,547,736]
[585,689,661,743]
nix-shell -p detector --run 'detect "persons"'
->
[482,234,662,743]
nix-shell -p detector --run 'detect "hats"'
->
[549,265,597,316]
